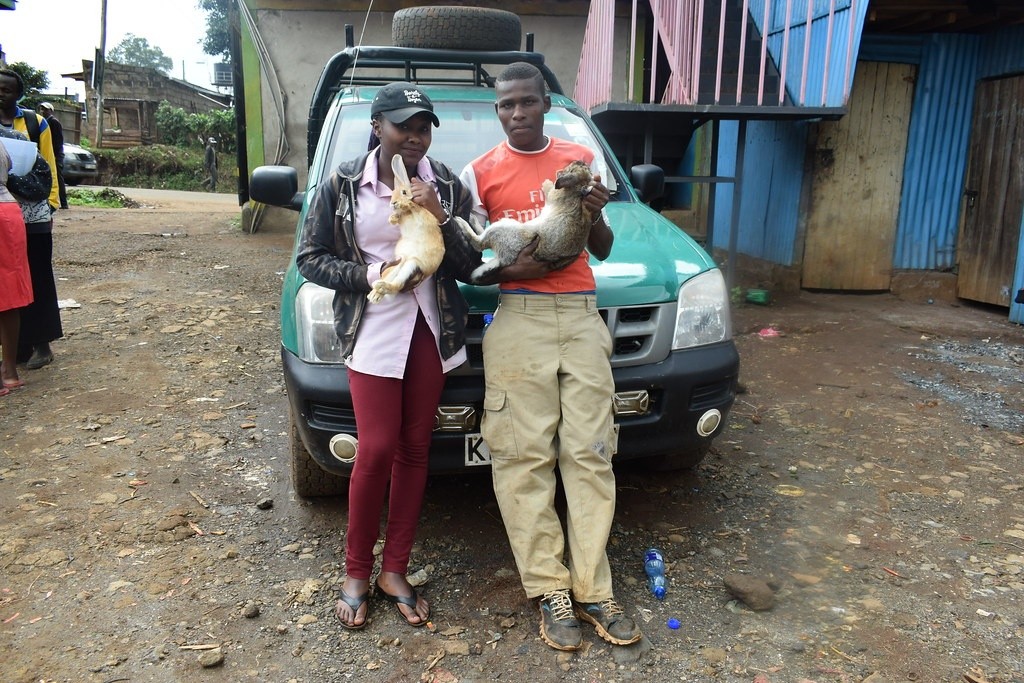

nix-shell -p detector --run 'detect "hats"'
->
[40,102,54,112]
[370,81,440,128]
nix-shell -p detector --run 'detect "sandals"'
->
[336,589,369,629]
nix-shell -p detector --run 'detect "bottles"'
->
[641,548,667,601]
[482,314,493,340]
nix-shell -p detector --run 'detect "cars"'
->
[62,142,99,186]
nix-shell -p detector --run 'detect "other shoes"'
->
[59,205,68,209]
[27,350,53,369]
[0,388,9,395]
[3,379,26,390]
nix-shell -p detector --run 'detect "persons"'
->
[458,61,643,650]
[296,81,486,631]
[0,67,69,396]
[200,137,222,194]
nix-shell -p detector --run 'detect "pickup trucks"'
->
[248,23,739,502]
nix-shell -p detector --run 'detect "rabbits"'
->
[366,153,446,304]
[453,159,595,281]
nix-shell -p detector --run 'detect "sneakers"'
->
[538,589,584,650]
[575,598,643,645]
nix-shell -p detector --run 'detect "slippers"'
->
[374,580,432,626]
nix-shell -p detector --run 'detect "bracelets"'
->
[593,211,603,226]
